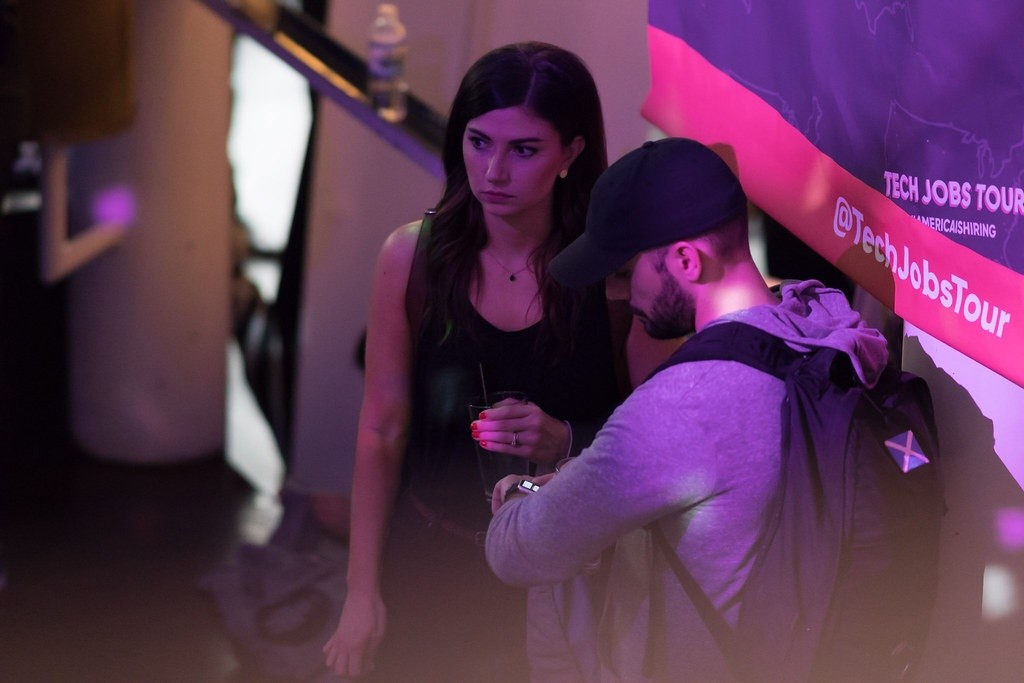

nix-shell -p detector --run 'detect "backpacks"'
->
[632,320,941,683]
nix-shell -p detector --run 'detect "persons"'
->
[317,41,629,683]
[483,137,899,683]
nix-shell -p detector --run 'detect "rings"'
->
[511,433,519,448]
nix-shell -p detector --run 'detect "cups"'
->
[467,390,529,506]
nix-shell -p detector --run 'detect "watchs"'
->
[504,480,540,503]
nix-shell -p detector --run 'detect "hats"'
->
[548,137,748,290]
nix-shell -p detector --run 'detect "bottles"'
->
[366,4,408,124]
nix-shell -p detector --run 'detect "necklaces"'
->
[487,250,535,281]
